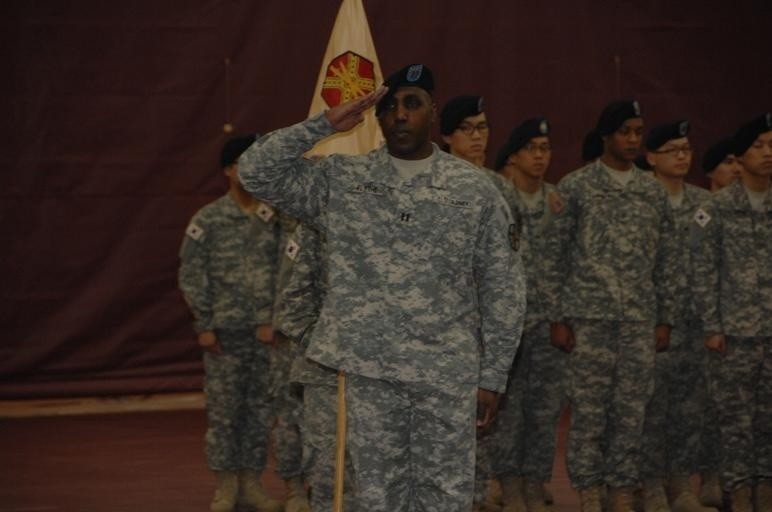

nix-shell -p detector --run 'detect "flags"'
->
[304,1,383,158]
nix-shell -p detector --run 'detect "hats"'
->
[702,136,735,172]
[581,128,605,161]
[596,99,641,134]
[375,63,436,115]
[439,94,483,135]
[504,117,549,156]
[219,131,263,168]
[734,112,772,157]
[645,117,688,151]
[496,142,510,170]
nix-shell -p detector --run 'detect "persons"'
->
[495,144,519,180]
[689,116,772,512]
[236,63,527,512]
[697,141,744,507]
[273,218,350,512]
[506,119,568,512]
[245,203,306,511]
[176,133,283,512]
[635,116,718,512]
[582,131,603,165]
[535,99,685,512]
[437,94,531,512]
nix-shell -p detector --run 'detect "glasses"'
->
[522,141,553,153]
[722,158,744,164]
[654,142,694,155]
[458,121,492,135]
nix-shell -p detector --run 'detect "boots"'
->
[671,488,718,512]
[525,481,552,512]
[697,470,723,506]
[284,476,310,512]
[499,475,525,512]
[579,485,601,512]
[609,486,635,512]
[211,470,239,512]
[544,487,553,503]
[238,468,283,512]
[725,486,753,512]
[754,483,772,512]
[642,478,669,512]
